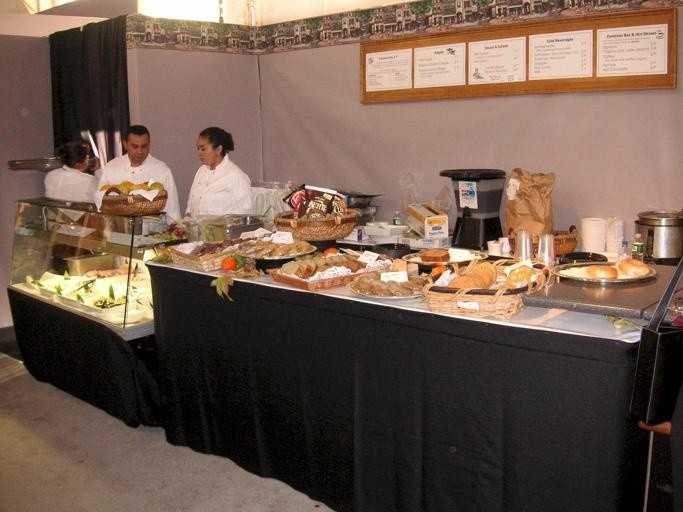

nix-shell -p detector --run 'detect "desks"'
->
[139,239,653,509]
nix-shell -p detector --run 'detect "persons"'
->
[94,125,181,237]
[184,127,253,218]
[43,139,98,255]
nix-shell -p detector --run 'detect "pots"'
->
[632,209,683,263]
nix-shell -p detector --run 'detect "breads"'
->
[619,259,651,276]
[589,265,617,279]
[238,237,541,297]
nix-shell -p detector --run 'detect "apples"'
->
[101,182,163,196]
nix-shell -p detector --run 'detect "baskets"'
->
[97,189,167,217]
[422,259,551,318]
[508,230,578,258]
[273,208,360,243]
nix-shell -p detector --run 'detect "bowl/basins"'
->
[374,242,410,259]
[552,231,576,256]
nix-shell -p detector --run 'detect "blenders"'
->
[438,168,507,251]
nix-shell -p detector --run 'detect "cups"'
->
[514,231,533,262]
[604,215,624,253]
[536,234,556,267]
[580,218,605,252]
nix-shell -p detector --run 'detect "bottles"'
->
[618,239,631,262]
[631,233,645,262]
[392,211,402,225]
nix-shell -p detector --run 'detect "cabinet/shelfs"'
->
[6,195,156,427]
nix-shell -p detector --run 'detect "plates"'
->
[347,276,421,300]
[559,251,607,265]
[550,262,654,283]
[236,244,317,261]
[402,247,488,269]
